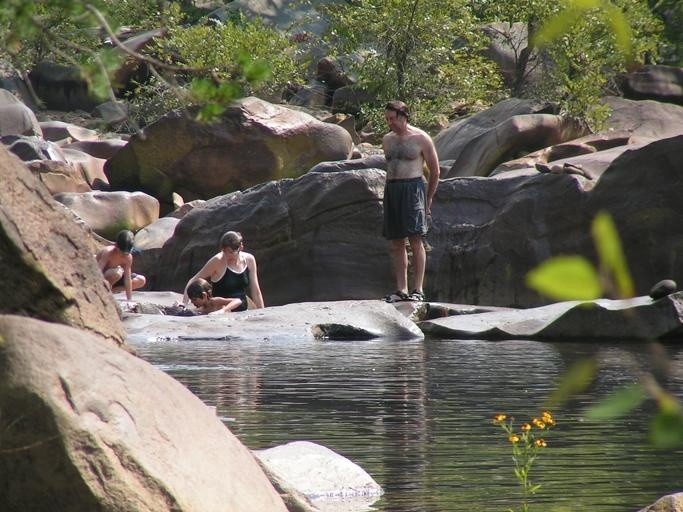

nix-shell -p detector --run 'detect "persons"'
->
[95,230,147,310]
[382,101,441,304]
[173,231,264,316]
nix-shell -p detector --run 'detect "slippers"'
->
[380,290,407,303]
[403,289,425,301]
